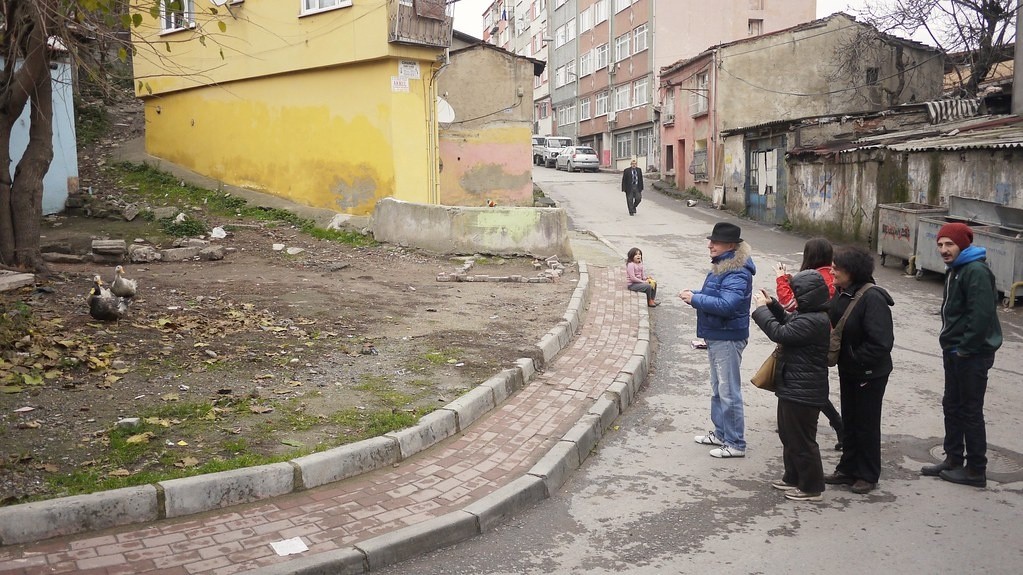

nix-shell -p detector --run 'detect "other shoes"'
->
[648,298,661,307]
[630,208,636,215]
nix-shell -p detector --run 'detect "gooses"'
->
[111,265,137,298]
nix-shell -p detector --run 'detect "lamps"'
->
[542,36,554,41]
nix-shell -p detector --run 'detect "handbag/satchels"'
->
[750,349,776,392]
[827,328,842,367]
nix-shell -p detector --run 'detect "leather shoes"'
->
[921,456,964,476]
[939,464,986,487]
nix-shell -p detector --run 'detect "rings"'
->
[779,267,781,270]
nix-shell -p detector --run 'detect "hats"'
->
[937,223,973,251]
[706,222,743,243]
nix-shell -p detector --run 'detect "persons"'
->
[622,160,643,215]
[679,222,756,457]
[824,247,894,495]
[773,238,845,450]
[626,248,661,307]
[921,222,1003,488]
[754,269,826,501]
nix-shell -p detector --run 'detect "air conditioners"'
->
[663,113,674,125]
[606,63,615,73]
[607,112,615,121]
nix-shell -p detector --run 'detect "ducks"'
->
[87,275,132,320]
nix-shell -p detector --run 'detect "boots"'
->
[829,413,844,450]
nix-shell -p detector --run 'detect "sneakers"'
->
[710,444,745,458]
[824,469,852,484]
[772,480,797,490]
[784,488,822,501]
[694,431,724,445]
[852,478,876,494]
